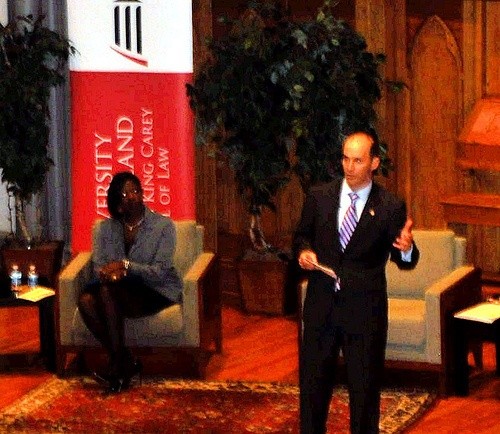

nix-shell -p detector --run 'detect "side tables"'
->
[444,292,500,396]
[0,275,57,374]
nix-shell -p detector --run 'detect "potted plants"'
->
[184,0,409,316]
[0,12,83,282]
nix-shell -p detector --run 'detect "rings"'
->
[112,275,116,281]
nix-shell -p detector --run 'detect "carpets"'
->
[0,372,436,434]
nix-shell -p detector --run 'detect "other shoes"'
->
[90,351,135,392]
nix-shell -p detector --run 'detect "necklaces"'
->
[121,217,144,232]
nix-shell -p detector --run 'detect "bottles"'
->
[8,264,22,292]
[26,265,39,290]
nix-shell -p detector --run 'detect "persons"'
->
[292,130,420,434]
[75,171,183,394]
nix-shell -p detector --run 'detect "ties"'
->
[339,193,359,253]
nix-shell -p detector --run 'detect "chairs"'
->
[295,228,482,397]
[54,217,221,381]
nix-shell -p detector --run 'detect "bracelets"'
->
[122,258,131,276]
